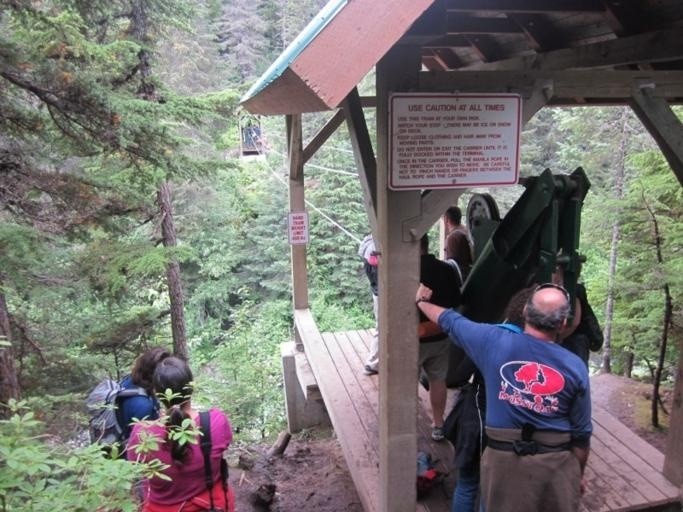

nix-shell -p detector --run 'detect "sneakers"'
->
[432,425,444,440]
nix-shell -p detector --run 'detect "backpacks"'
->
[86,378,148,446]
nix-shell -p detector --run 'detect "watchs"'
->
[416,296,428,304]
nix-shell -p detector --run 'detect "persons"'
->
[453,290,531,512]
[357,231,379,376]
[444,206,472,270]
[112,345,173,462]
[123,355,237,512]
[419,233,461,440]
[566,285,604,368]
[417,282,592,512]
